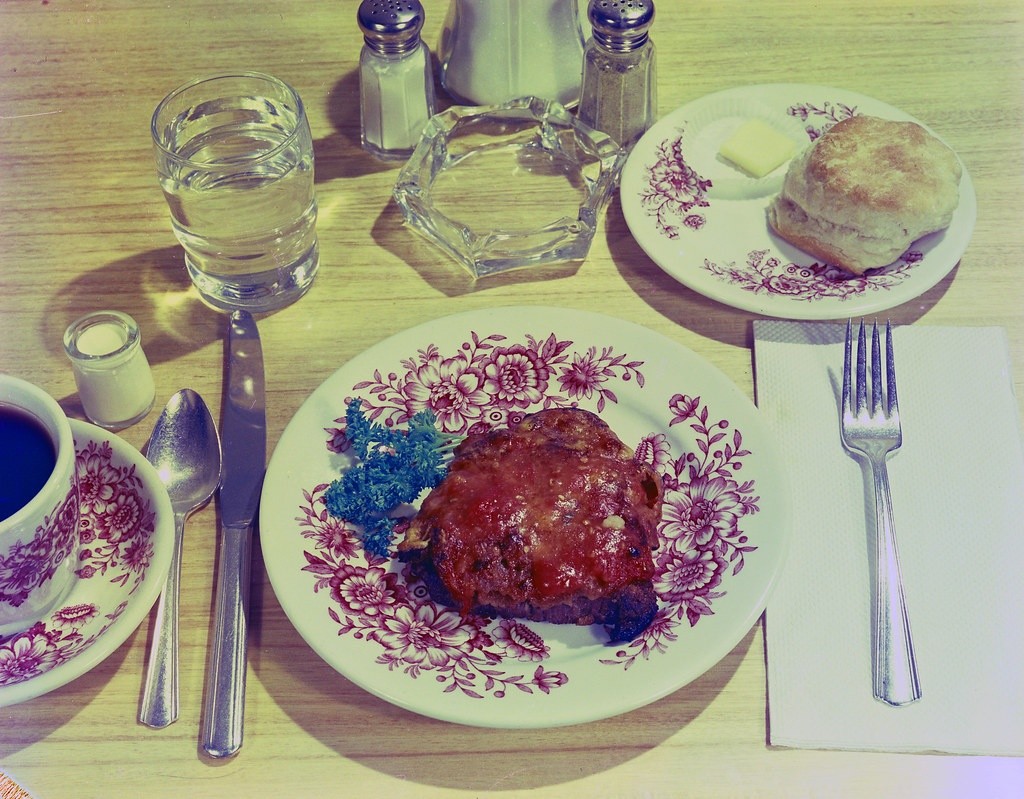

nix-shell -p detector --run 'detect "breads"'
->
[768,114,963,274]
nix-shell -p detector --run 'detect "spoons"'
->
[138,387,221,728]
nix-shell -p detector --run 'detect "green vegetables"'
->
[322,397,471,557]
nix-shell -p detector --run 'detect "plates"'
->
[260,306,793,728]
[619,83,977,320]
[0,418,175,707]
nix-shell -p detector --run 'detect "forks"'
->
[842,316,922,706]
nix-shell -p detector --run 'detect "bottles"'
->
[356,0,433,160]
[578,0,658,188]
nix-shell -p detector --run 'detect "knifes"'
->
[200,311,267,758]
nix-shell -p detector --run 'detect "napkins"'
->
[751,319,1024,757]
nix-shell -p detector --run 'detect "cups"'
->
[435,0,588,115]
[151,71,323,314]
[64,311,156,429]
[0,375,79,624]
